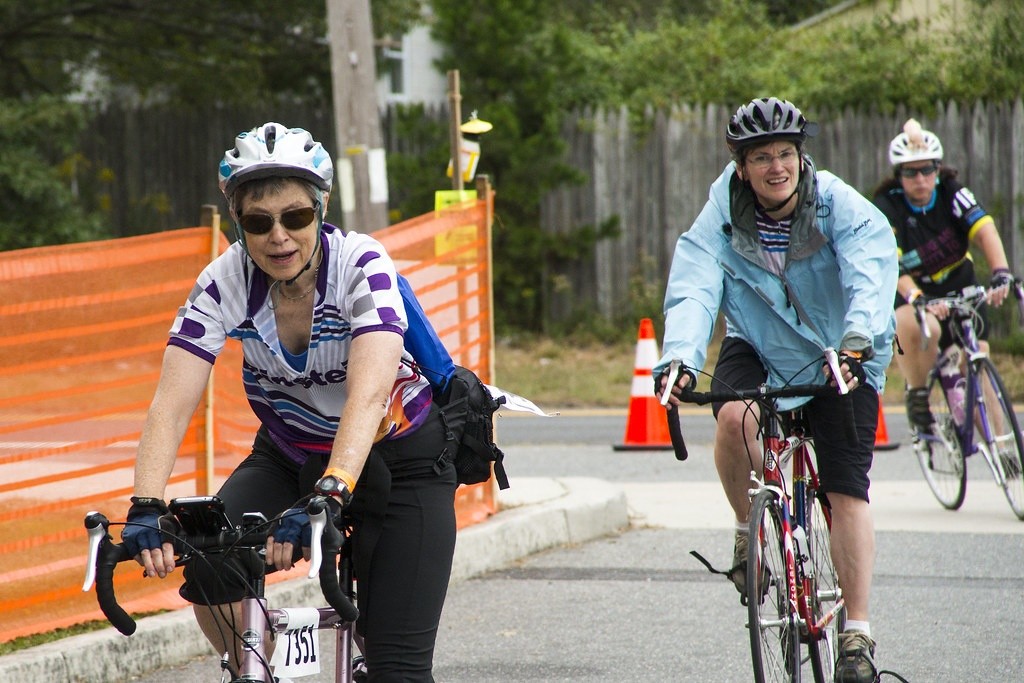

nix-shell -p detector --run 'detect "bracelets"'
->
[905,288,922,304]
[852,351,861,358]
[322,468,356,494]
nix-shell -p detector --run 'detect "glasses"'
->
[897,160,937,177]
[235,201,324,234]
[743,150,803,169]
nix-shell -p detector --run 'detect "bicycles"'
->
[654,345,878,683]
[81,491,368,683]
[902,276,1024,524]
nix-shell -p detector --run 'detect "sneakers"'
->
[905,387,933,425]
[834,629,877,683]
[993,456,1021,478]
[729,529,770,596]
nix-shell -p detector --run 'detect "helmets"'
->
[889,118,944,165]
[219,121,333,199]
[727,97,805,146]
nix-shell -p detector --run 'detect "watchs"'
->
[315,475,353,505]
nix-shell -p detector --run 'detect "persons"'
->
[120,122,457,683]
[652,96,899,683]
[872,118,1021,479]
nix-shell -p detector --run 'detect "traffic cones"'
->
[871,393,901,452]
[612,316,676,452]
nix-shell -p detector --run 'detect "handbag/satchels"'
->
[338,234,457,393]
[433,365,511,490]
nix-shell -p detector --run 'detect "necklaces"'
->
[276,251,323,301]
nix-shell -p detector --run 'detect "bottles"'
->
[790,515,812,574]
[940,364,966,426]
